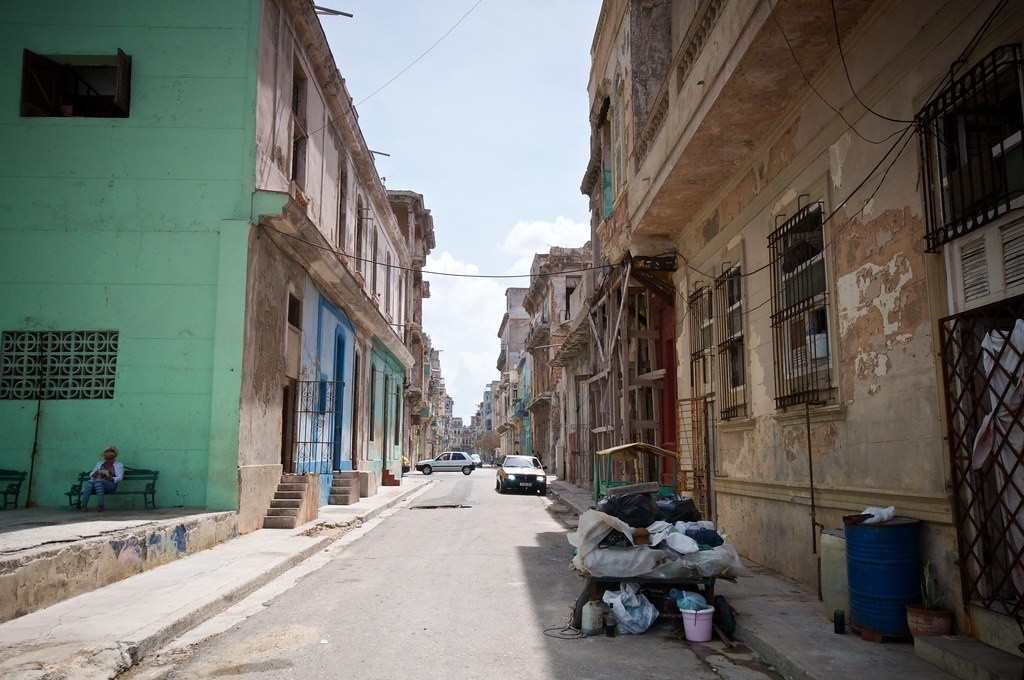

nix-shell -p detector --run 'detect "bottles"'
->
[581,600,603,635]
[606,610,614,636]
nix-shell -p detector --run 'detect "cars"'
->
[469,454,483,468]
[496,455,549,496]
[402,456,410,474]
[416,450,475,475]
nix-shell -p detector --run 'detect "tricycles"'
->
[572,442,736,618]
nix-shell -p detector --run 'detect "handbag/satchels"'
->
[685,527,724,546]
[598,531,629,549]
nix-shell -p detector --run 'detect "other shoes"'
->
[92,505,104,512]
[82,505,87,512]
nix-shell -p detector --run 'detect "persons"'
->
[532,450,541,463]
[513,451,519,455]
[439,454,450,460]
[81,446,123,512]
[489,454,494,464]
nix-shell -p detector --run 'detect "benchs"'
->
[64,469,160,511]
[0,467,28,511]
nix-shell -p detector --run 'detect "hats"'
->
[632,528,650,545]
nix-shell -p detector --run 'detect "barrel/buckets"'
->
[843,514,925,638]
[679,605,715,642]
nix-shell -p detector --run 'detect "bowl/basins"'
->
[645,593,682,631]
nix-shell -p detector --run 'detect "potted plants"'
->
[907,561,955,637]
